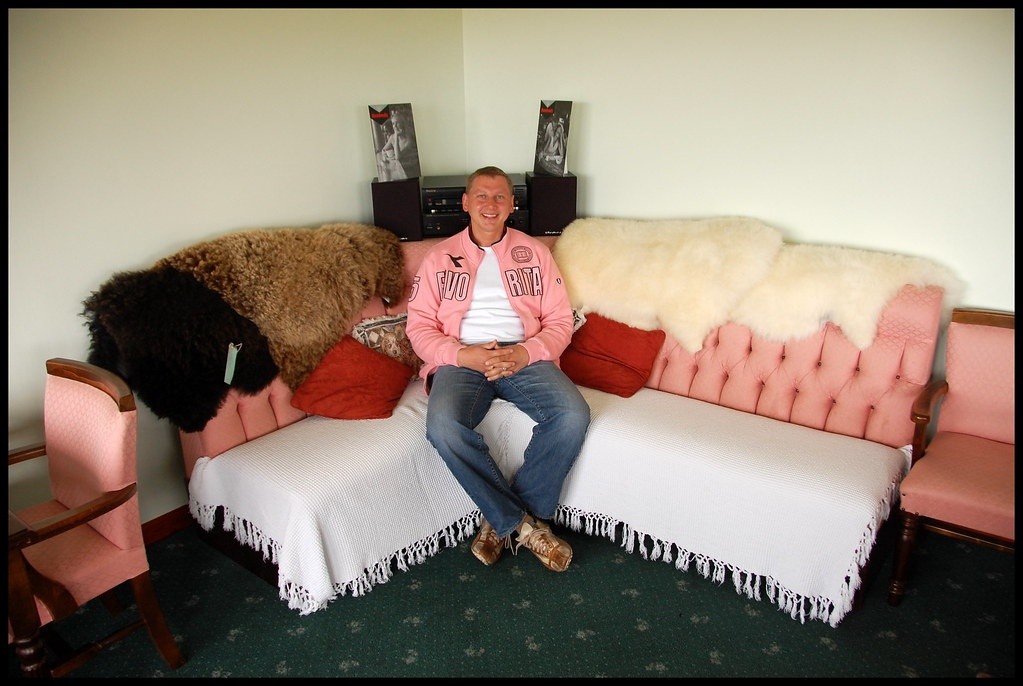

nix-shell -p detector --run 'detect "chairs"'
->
[888,306,1015,605]
[6,357,186,683]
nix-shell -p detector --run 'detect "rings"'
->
[503,368,506,371]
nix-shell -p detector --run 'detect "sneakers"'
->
[471,515,515,567]
[514,518,573,572]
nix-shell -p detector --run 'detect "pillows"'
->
[352,314,423,379]
[289,333,412,418]
[560,311,668,398]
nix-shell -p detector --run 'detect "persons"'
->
[406,166,591,572]
[540,116,565,161]
[382,114,420,177]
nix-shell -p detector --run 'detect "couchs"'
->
[175,229,943,582]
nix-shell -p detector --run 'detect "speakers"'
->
[371,176,424,243]
[525,171,578,237]
